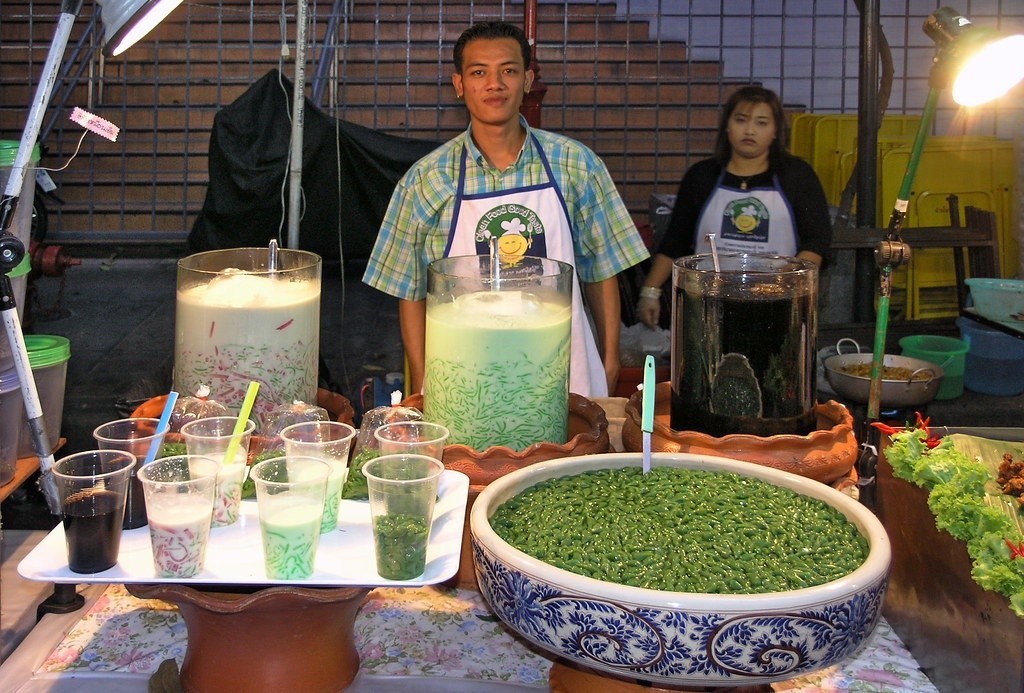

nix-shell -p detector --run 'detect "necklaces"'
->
[731,160,764,189]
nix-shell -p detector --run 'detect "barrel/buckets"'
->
[954,307,1024,398]
[898,334,969,401]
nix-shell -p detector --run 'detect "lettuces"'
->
[884,429,1024,622]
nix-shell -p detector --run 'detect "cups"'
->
[52,450,137,573]
[92,417,171,530]
[138,454,221,579]
[422,255,573,451]
[280,422,356,533]
[250,456,332,580]
[181,416,256,526]
[374,422,450,514]
[0,139,72,487]
[669,252,818,439]
[362,454,445,579]
[174,246,323,441]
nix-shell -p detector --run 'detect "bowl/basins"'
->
[470,451,891,687]
[622,381,857,484]
[387,392,617,488]
[824,337,946,407]
[964,277,1024,323]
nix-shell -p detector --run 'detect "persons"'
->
[638,85,833,328]
[362,21,651,400]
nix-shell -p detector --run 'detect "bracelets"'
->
[640,287,662,299]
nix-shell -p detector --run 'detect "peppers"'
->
[870,411,940,449]
[1011,552,1018,559]
[1018,541,1024,557]
[1005,538,1021,556]
